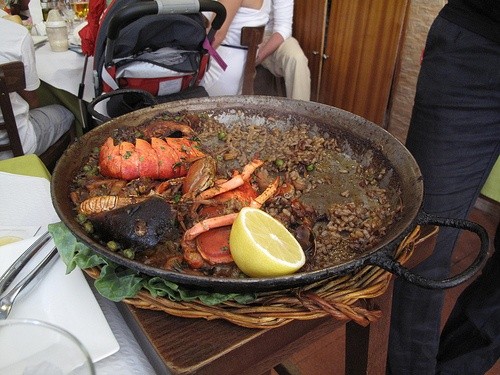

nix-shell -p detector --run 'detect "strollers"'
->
[77,0,227,133]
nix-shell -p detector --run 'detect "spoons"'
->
[0,230,52,297]
[0,245,59,319]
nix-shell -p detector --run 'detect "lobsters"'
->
[98,135,279,269]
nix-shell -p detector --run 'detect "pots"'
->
[50,87,490,290]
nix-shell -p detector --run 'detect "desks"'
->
[114,299,379,375]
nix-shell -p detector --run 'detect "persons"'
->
[195,0,264,97]
[254,0,311,101]
[0,0,75,162]
[387,0,500,374]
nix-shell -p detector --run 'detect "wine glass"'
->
[69,0,90,40]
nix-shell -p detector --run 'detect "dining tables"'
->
[2,155,158,375]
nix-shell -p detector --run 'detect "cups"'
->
[0,320,96,375]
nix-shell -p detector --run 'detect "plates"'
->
[0,234,121,374]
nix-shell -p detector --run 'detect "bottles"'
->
[44,9,70,52]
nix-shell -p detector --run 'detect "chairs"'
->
[240,25,265,95]
[0,61,27,157]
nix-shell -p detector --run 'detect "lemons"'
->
[230,207,305,275]
